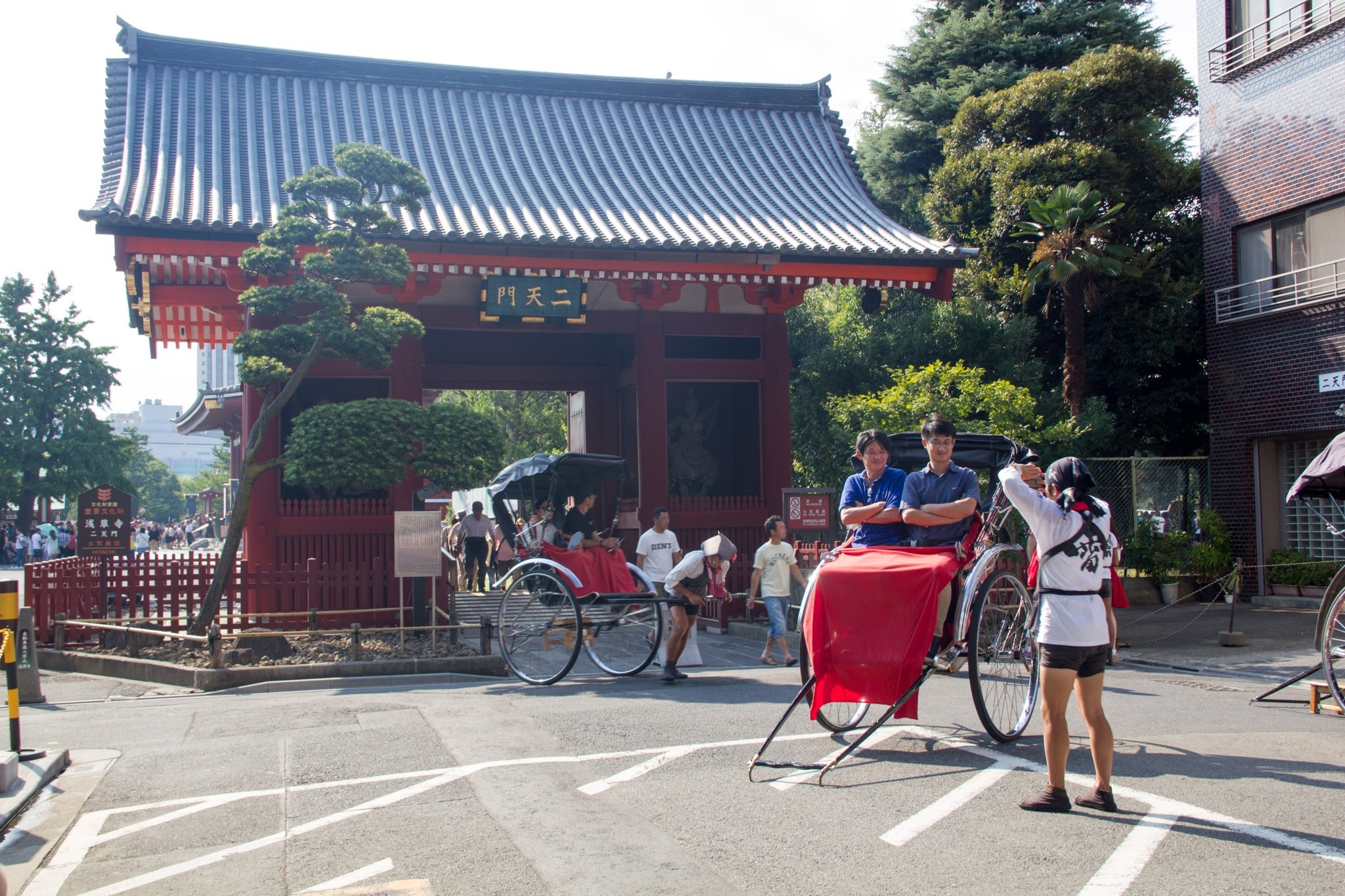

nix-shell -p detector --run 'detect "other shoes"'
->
[1111,649,1118,666]
[1017,783,1071,811]
[477,579,484,591]
[468,579,473,590]
[924,645,941,662]
[643,634,653,649]
[674,671,688,678]
[661,670,675,682]
[1076,784,1117,811]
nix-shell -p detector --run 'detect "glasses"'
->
[592,494,598,499]
[926,438,953,448]
[539,506,554,512]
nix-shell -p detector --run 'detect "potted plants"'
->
[1125,504,1234,605]
[1266,546,1341,598]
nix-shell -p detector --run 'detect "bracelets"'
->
[1008,461,1022,475]
[599,540,603,546]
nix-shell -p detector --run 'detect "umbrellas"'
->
[35,523,59,538]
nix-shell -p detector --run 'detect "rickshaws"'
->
[1248,429,1345,716]
[486,449,751,687]
[746,429,1041,787]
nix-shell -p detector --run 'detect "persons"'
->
[0,520,77,569]
[838,429,908,548]
[898,414,981,659]
[522,499,583,553]
[1024,505,1127,667]
[746,514,808,667]
[441,501,528,592]
[661,531,737,681]
[636,508,683,651]
[130,511,219,563]
[563,484,622,552]
[997,455,1118,814]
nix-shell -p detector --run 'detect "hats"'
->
[516,517,526,525]
[456,510,467,519]
[471,501,484,509]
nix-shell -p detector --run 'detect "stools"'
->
[1310,681,1345,714]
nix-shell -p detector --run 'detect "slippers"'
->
[760,656,777,665]
[785,657,798,666]
